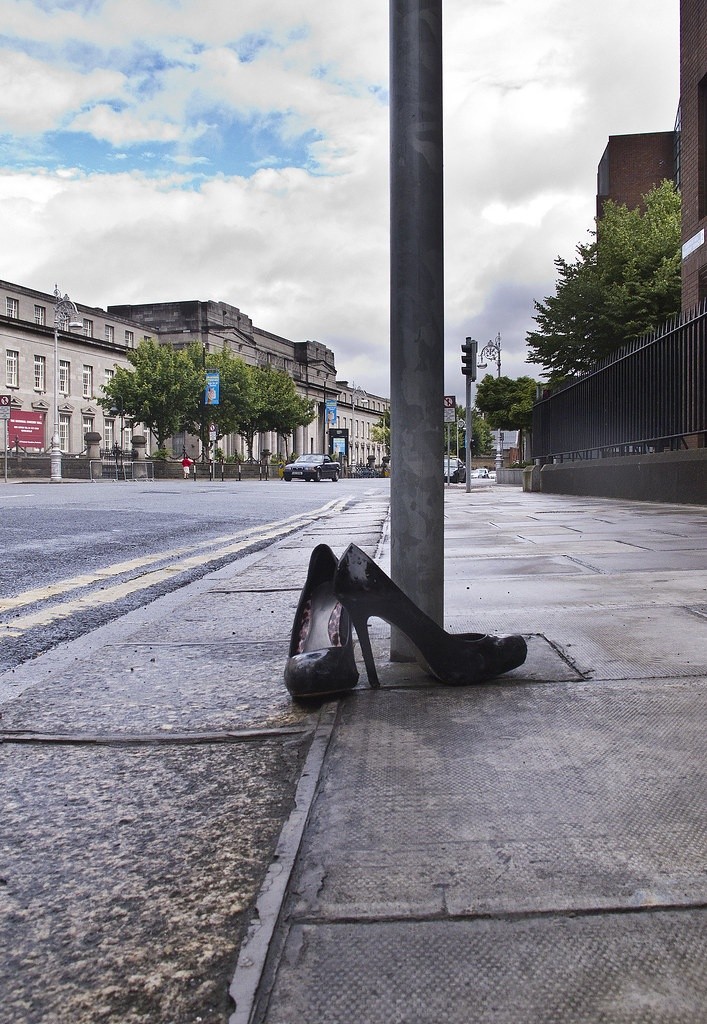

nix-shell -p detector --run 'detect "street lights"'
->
[455,418,466,483]
[351,388,369,473]
[109,395,124,480]
[49,295,82,481]
[476,341,502,482]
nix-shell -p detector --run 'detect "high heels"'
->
[285,544,360,699]
[333,542,528,688]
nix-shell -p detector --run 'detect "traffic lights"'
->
[500,433,504,440]
[462,341,477,381]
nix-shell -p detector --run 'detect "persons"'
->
[182,457,191,479]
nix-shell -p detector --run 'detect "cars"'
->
[471,469,496,479]
[283,453,340,481]
[444,455,466,483]
